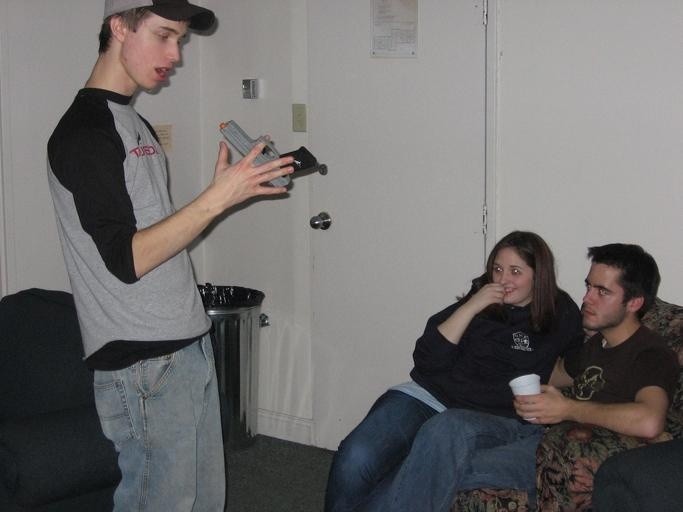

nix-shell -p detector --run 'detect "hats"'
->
[103,0,219,36]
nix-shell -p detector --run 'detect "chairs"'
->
[453,296,683,512]
[0,289,122,512]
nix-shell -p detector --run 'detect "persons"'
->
[322,230,588,511]
[377,243,680,511]
[41,0,296,511]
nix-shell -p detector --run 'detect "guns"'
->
[219,120,317,186]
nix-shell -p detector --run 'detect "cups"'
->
[508,374,541,421]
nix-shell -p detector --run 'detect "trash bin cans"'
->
[198,283,265,447]
